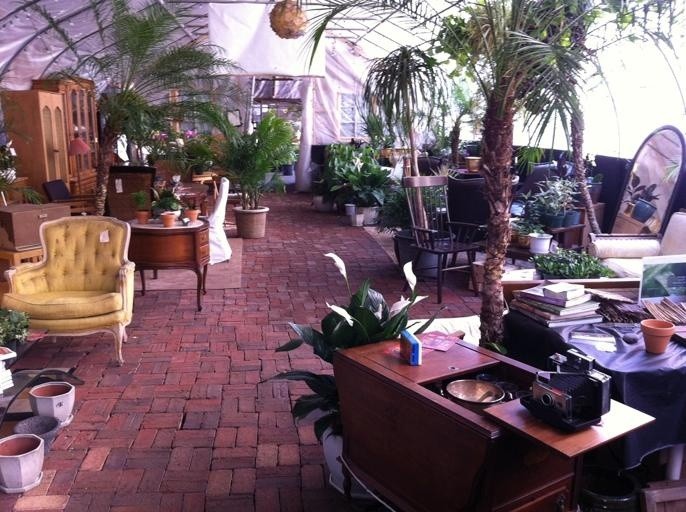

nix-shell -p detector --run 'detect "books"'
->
[0,346,18,395]
[509,280,604,328]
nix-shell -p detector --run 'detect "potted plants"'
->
[196,109,300,238]
[623,173,660,224]
[508,174,603,254]
[257,251,448,496]
[310,141,391,227]
[131,188,201,228]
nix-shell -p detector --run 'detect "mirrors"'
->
[608,125,686,234]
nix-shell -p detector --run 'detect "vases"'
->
[465,157,481,172]
[639,319,676,353]
[0,382,76,494]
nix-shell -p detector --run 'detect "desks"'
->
[126,217,209,312]
[501,295,686,482]
[332,330,657,512]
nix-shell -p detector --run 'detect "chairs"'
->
[2,216,137,365]
[399,175,484,305]
[588,212,686,280]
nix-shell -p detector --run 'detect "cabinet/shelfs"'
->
[1,78,100,195]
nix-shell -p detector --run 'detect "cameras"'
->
[532,361,612,424]
